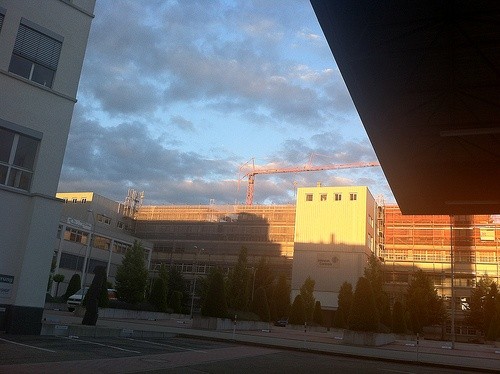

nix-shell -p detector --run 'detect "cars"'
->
[274,319,287,327]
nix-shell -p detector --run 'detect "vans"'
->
[67,287,117,311]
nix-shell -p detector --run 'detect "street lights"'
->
[188,244,205,319]
[251,265,259,300]
[81,208,106,307]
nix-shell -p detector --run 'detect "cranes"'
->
[240,157,380,204]
